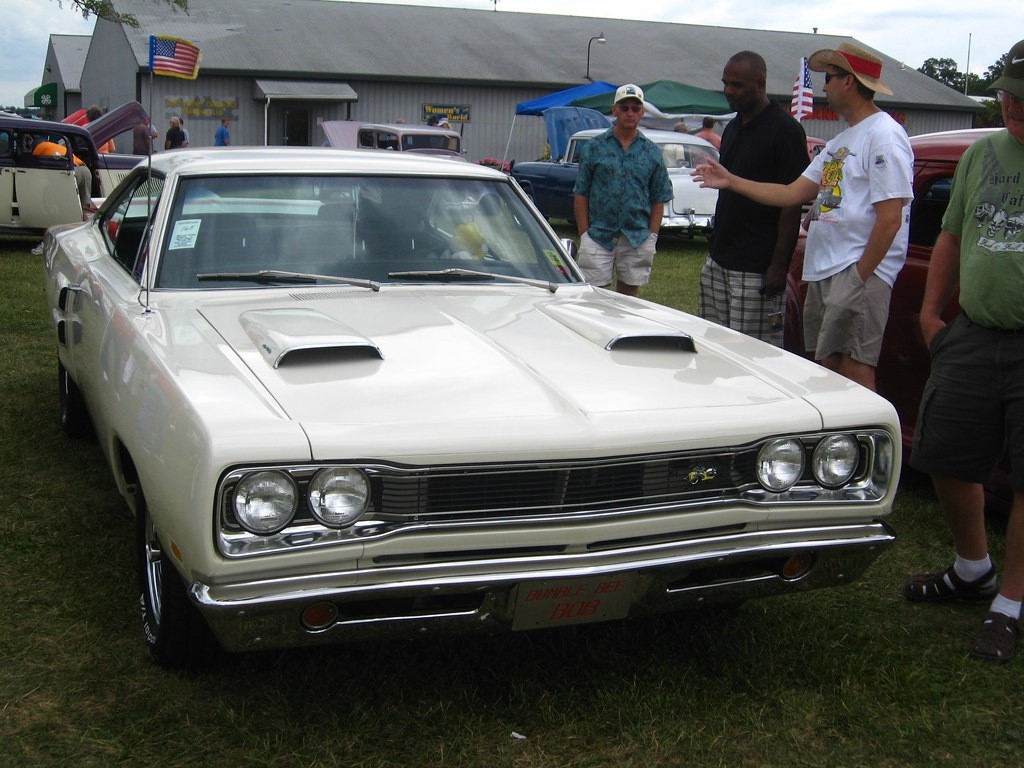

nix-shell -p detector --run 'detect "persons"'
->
[214,118,230,146]
[573,84,675,297]
[86,107,116,154]
[902,40,1024,663]
[165,116,189,150]
[132,116,158,155]
[663,116,721,169]
[689,42,915,397]
[426,116,456,151]
[32,138,99,223]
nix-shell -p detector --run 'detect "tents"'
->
[501,80,619,173]
[565,80,732,116]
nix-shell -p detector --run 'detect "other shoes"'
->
[31,242,43,255]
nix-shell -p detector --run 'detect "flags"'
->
[792,57,813,124]
[149,35,201,81]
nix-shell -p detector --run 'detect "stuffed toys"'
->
[427,224,489,263]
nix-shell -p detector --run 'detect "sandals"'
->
[972,612,1022,661]
[904,559,1000,603]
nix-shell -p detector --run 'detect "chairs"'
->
[353,213,427,259]
[180,214,269,268]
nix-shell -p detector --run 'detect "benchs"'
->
[116,212,406,266]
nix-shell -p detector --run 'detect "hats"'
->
[809,42,894,96]
[986,39,1024,101]
[615,83,644,104]
[438,118,452,129]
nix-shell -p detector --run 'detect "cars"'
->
[783,126,1005,446]
[805,137,832,162]
[42,144,903,661]
[0,99,158,236]
[512,125,721,232]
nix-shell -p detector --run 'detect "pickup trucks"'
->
[319,121,499,216]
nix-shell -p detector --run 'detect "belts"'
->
[74,163,86,167]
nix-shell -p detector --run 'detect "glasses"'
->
[618,105,642,113]
[825,72,850,84]
[996,90,1024,105]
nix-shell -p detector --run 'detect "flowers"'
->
[474,157,511,172]
